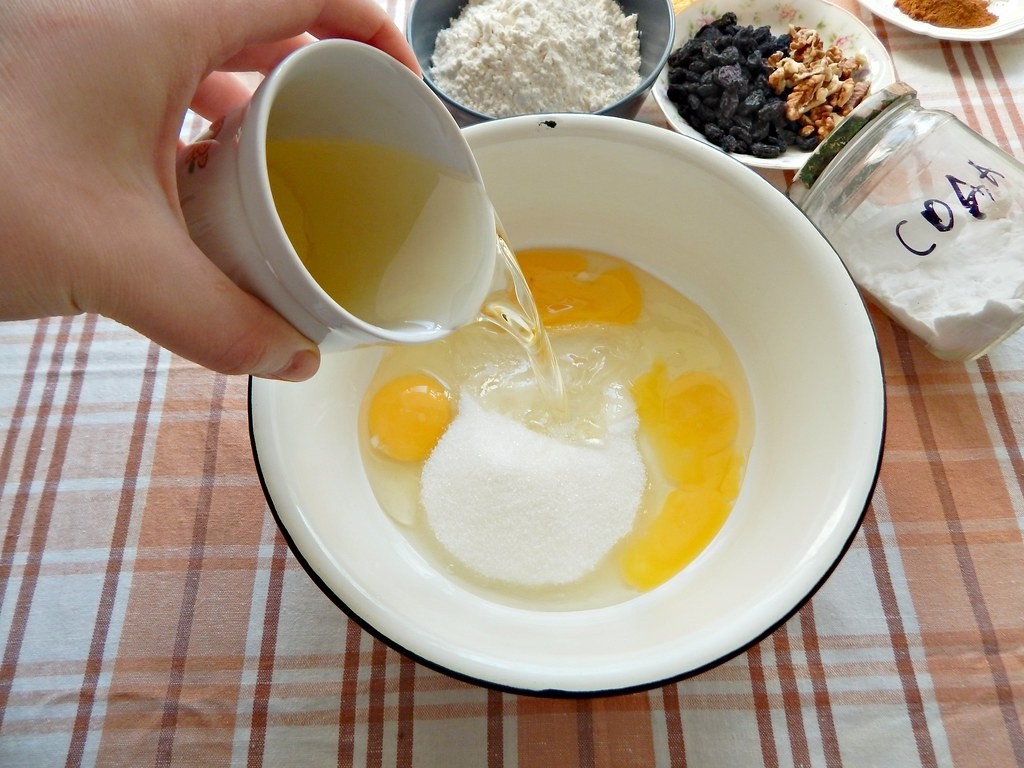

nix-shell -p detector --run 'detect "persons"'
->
[0,0,423,382]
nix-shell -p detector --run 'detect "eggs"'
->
[364,373,454,464]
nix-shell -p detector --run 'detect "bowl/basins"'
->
[246,114,889,698]
[408,0,675,119]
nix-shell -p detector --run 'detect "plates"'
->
[859,0,1024,41]
[651,0,896,169]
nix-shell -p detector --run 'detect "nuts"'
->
[767,24,870,148]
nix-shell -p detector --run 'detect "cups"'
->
[785,82,1024,359]
[179,40,495,350]
